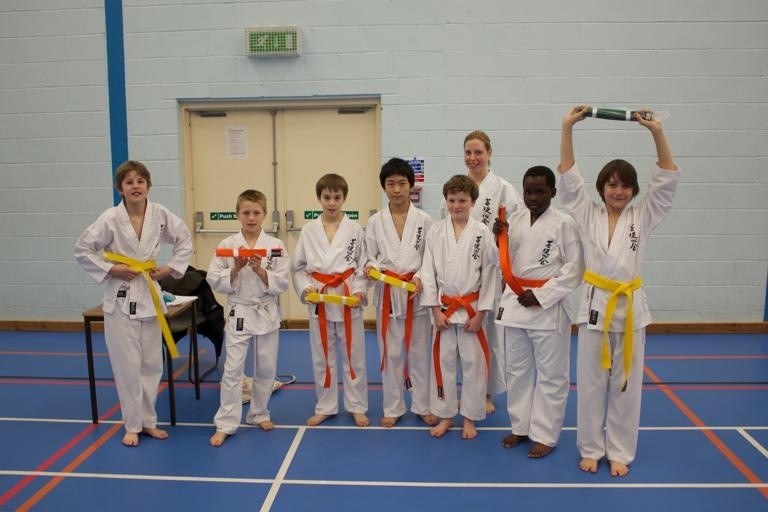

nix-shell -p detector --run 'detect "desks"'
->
[82,295,201,426]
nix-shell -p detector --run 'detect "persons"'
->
[440,130,526,414]
[206,190,290,446]
[291,174,368,427]
[422,175,497,439]
[493,166,584,458]
[365,159,440,427]
[74,160,193,447]
[556,104,682,477]
